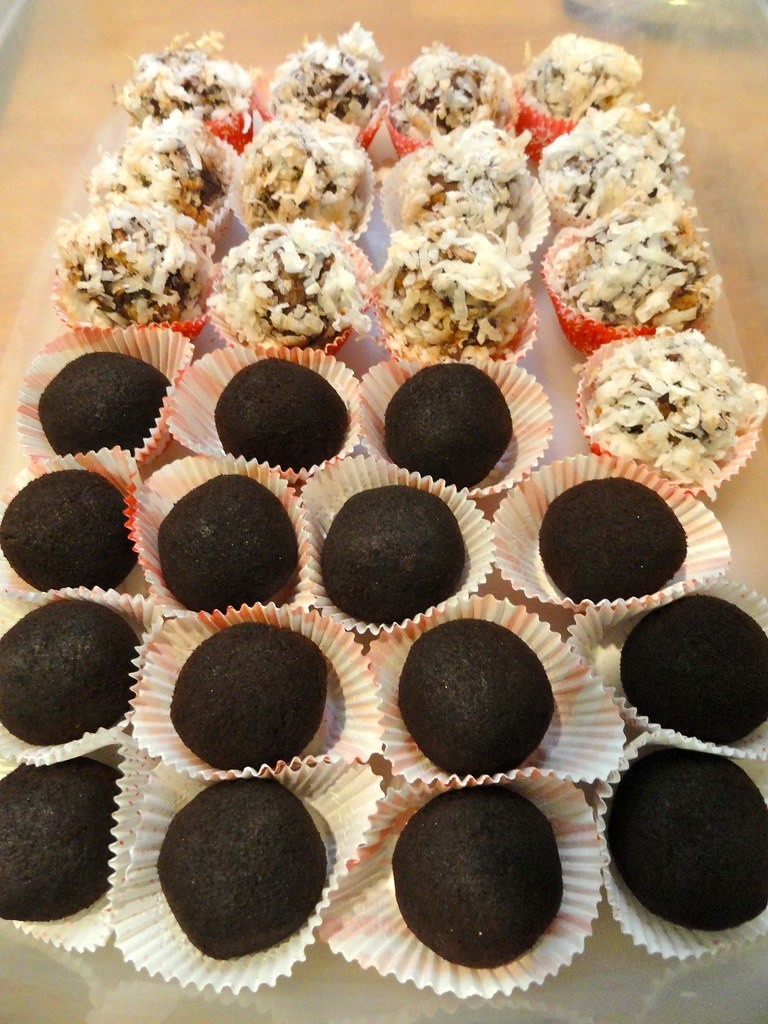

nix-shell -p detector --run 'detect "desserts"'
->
[1,22,768,999]
